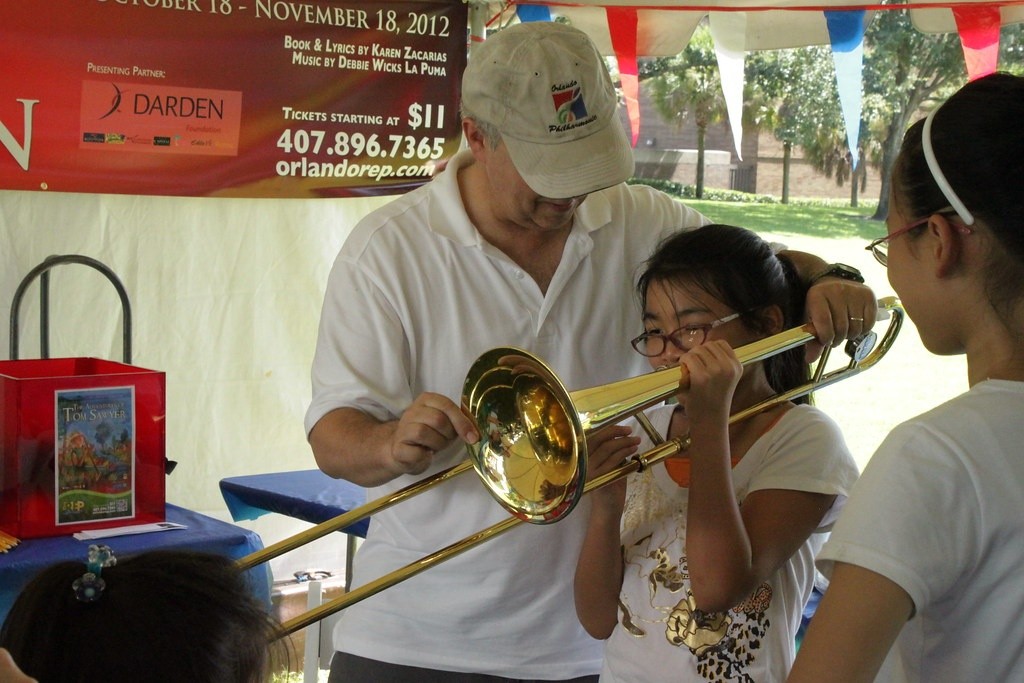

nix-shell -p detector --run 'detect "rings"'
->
[850,316,867,323]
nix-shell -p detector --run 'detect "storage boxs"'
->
[0,357,166,539]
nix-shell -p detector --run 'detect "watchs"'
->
[802,261,867,297]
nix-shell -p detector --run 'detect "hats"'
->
[458,20,638,202]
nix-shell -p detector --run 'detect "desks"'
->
[0,502,273,626]
[218,469,370,596]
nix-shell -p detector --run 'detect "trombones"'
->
[224,293,906,643]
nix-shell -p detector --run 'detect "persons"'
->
[571,222,861,683]
[788,72,1023,683]
[1,550,296,683]
[303,20,880,683]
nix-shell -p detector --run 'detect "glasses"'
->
[629,304,766,359]
[865,205,971,269]
